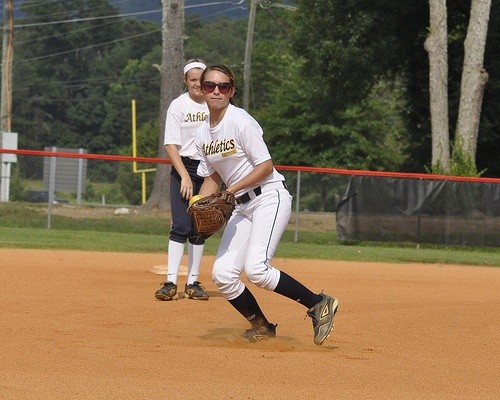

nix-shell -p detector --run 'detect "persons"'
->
[187,64,339,345]
[155,58,210,300]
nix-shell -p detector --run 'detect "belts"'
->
[235,180,287,205]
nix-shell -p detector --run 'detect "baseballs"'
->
[189,195,204,206]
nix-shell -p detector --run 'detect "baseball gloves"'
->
[186,189,236,241]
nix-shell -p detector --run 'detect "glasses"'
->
[201,81,233,94]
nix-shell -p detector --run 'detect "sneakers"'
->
[303,288,339,345]
[183,281,209,300]
[155,280,178,301]
[242,323,278,344]
